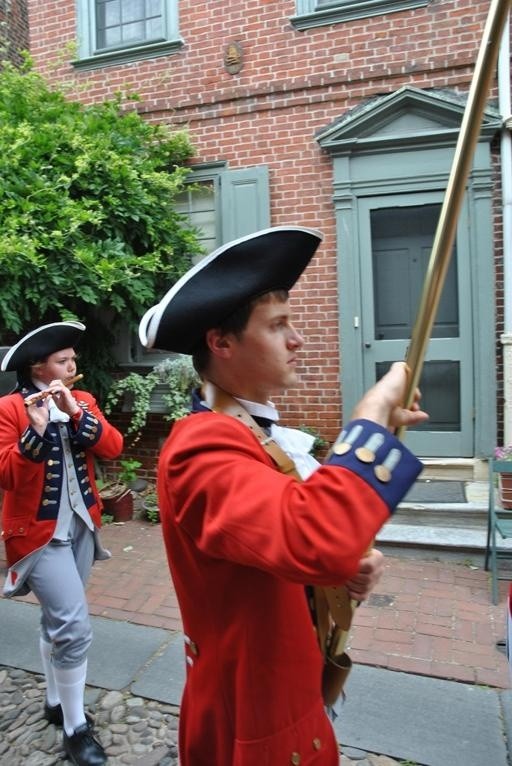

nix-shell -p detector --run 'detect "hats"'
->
[0,321,86,373]
[136,225,322,356]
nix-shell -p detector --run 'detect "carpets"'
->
[399,477,468,503]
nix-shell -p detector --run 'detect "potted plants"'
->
[97,457,160,523]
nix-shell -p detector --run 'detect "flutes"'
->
[24,374,83,406]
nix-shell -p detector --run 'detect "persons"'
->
[0,318,124,766]
[138,225,431,766]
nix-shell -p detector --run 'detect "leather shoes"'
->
[64,725,106,766]
[45,701,94,729]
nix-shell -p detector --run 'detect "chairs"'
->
[484,457,512,606]
